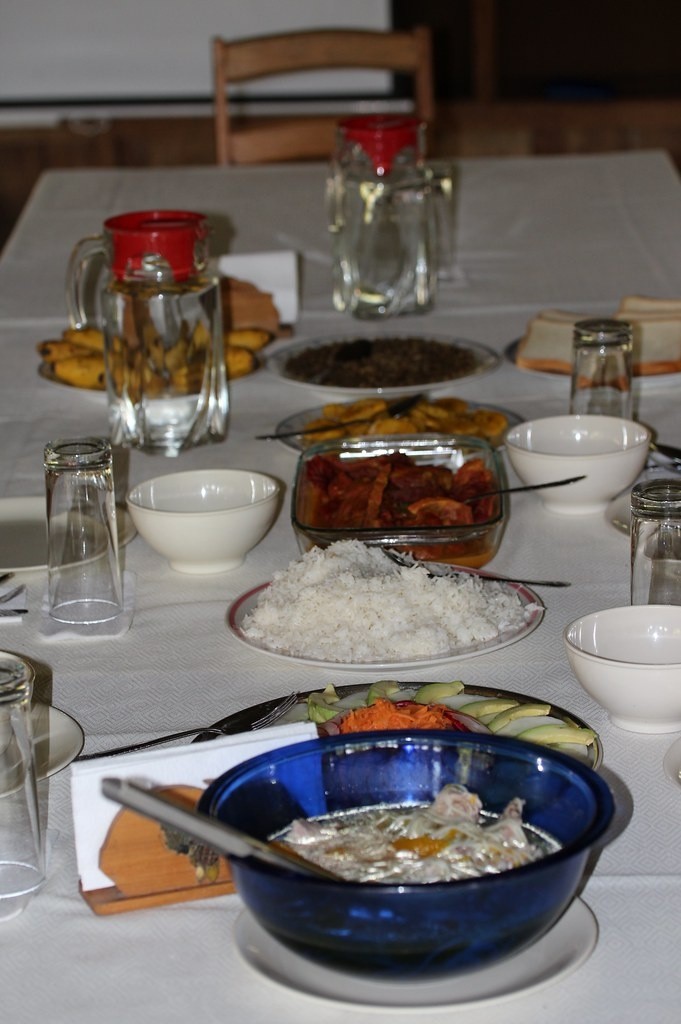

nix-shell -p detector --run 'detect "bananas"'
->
[36,322,271,390]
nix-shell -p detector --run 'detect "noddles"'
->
[265,783,563,884]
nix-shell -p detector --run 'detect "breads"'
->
[516,293,681,383]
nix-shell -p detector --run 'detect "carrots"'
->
[338,697,453,733]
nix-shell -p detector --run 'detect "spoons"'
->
[0,584,26,603]
[380,545,572,589]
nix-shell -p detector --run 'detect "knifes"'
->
[0,609,28,618]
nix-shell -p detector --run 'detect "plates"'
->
[0,702,85,798]
[34,356,257,402]
[0,496,136,573]
[261,330,501,400]
[233,895,599,1014]
[274,402,523,457]
[188,681,604,775]
[502,338,681,387]
[223,560,546,671]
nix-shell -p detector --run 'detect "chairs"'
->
[210,21,435,173]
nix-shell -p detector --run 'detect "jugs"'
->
[329,108,455,323]
[64,208,231,457]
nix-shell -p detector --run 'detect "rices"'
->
[237,539,548,662]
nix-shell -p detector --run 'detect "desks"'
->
[0,155,681,1024]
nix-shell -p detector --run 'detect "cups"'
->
[44,436,124,625]
[630,478,681,606]
[568,318,633,420]
[0,650,47,899]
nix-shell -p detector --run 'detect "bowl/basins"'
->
[197,730,616,988]
[125,468,280,576]
[561,604,681,734]
[290,434,510,570]
[504,413,652,514]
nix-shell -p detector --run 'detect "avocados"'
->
[456,698,598,747]
[367,680,400,706]
[307,684,344,724]
[413,680,463,704]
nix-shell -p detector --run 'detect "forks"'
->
[72,692,298,764]
